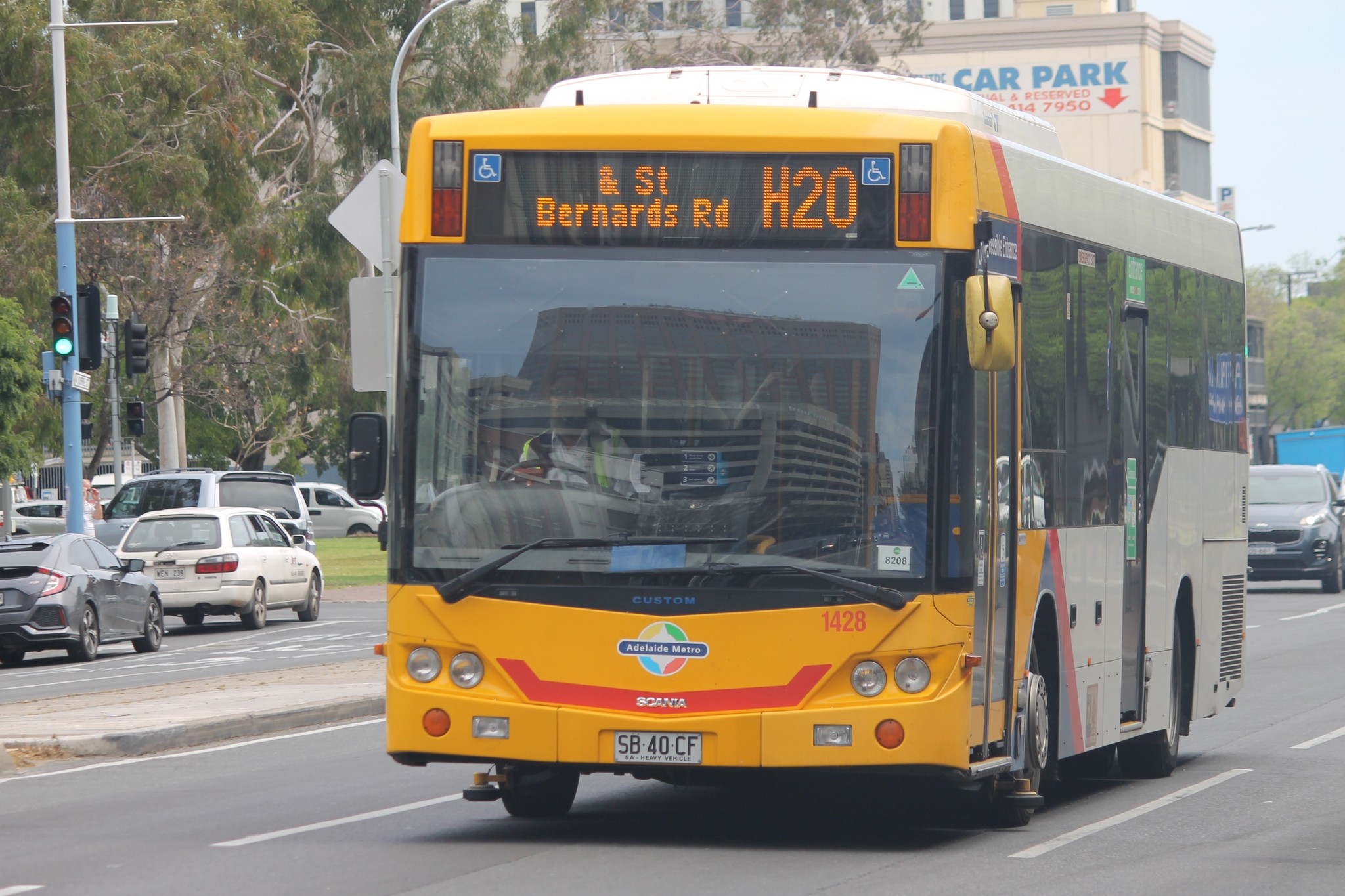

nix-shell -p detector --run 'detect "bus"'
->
[339,57,1255,826]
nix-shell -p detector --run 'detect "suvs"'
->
[94,465,318,557]
[1248,461,1345,595]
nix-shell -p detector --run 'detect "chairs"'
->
[154,524,175,548]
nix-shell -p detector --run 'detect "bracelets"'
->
[94,498,101,503]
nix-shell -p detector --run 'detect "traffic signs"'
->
[679,450,719,487]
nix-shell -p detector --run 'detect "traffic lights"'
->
[50,295,74,358]
[125,319,149,378]
[81,401,93,419]
[126,400,145,435]
[82,422,93,440]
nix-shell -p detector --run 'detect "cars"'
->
[0,464,331,541]
[0,532,164,667]
[113,505,325,630]
[982,455,1051,532]
[296,481,388,538]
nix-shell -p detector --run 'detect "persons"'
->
[62,479,103,538]
[520,367,641,499]
